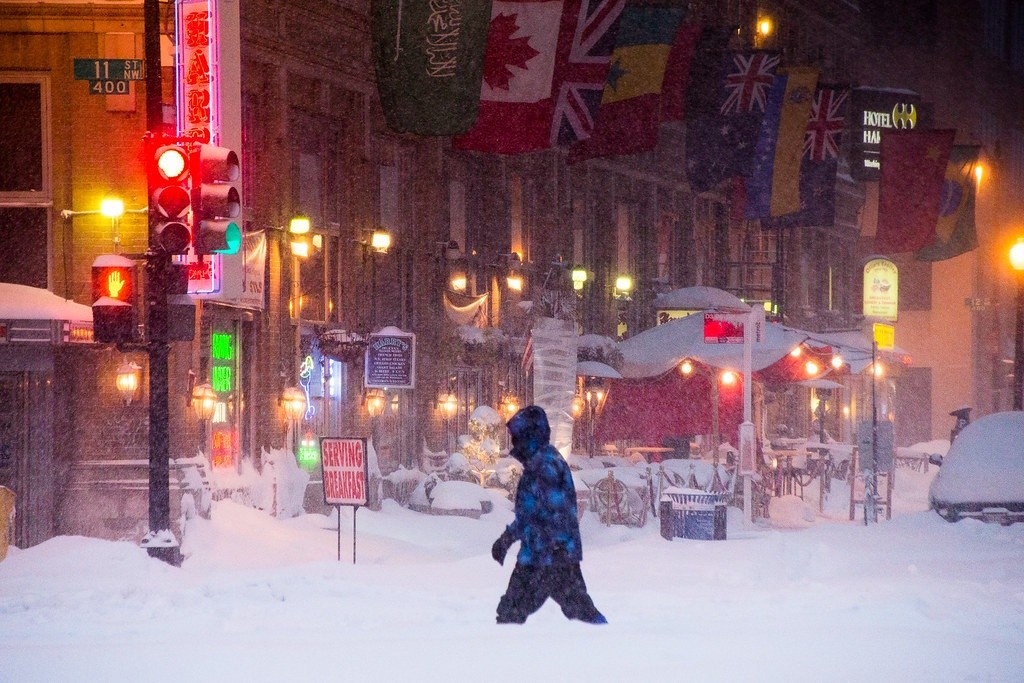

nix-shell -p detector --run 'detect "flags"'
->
[370,0,987,261]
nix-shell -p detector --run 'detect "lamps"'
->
[497,395,519,421]
[499,252,521,270]
[278,388,306,421]
[437,240,461,260]
[116,356,138,406]
[361,388,386,417]
[573,385,585,418]
[434,388,458,419]
[187,372,217,423]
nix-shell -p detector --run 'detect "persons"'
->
[949,407,971,446]
[490,404,608,626]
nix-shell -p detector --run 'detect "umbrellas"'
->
[611,302,879,467]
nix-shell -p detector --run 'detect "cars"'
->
[926,409,1024,527]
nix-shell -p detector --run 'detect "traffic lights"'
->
[190,140,242,258]
[143,136,190,255]
[88,254,139,341]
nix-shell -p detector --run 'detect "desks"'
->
[774,450,811,492]
[625,447,673,464]
[784,438,806,450]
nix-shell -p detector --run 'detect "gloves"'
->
[492,530,514,566]
